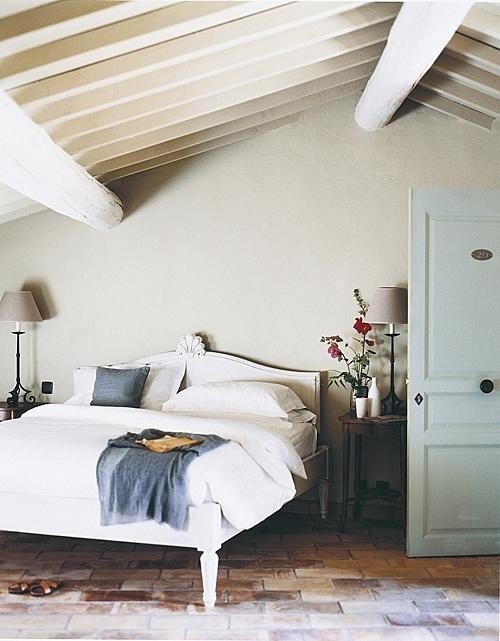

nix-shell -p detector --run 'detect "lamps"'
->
[0,289,44,405]
[362,287,407,414]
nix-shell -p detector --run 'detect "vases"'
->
[349,386,368,418]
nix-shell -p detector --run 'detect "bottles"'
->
[367,376,381,418]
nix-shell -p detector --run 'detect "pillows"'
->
[62,358,186,411]
[89,365,150,408]
[161,381,308,419]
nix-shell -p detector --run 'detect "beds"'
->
[0,333,335,614]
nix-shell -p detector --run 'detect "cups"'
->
[356,397,368,418]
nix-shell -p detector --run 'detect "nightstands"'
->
[337,412,407,551]
[0,402,43,421]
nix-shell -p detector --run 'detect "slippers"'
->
[8,579,43,593]
[31,580,62,596]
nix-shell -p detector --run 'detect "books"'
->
[134,434,205,454]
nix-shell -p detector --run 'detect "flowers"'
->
[318,288,377,398]
